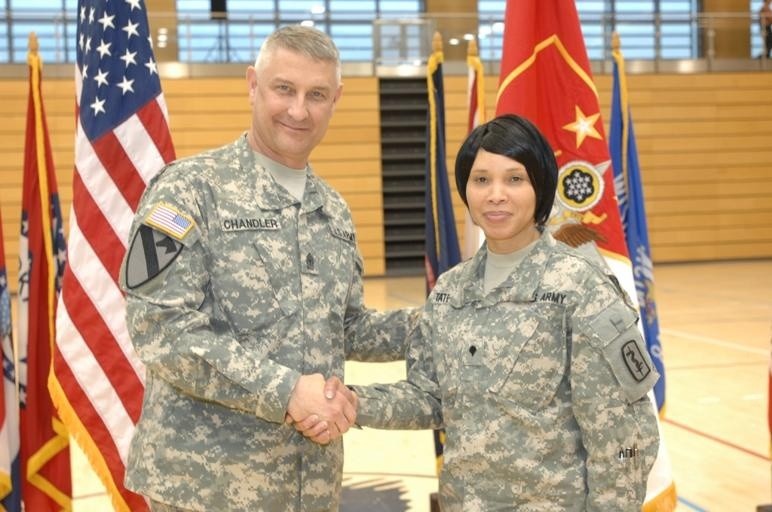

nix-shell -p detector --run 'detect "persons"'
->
[287,112,659,512]
[118,25,430,510]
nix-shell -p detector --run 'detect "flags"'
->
[0,217,21,511]
[460,55,490,262]
[610,50,665,415]
[425,53,463,475]
[53,0,180,511]
[19,52,71,512]
[480,1,677,511]
